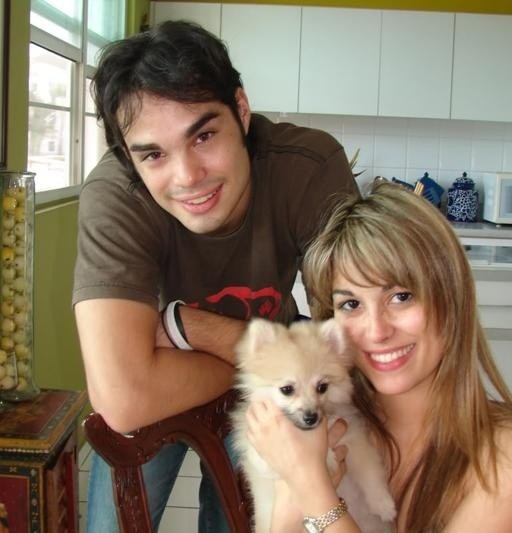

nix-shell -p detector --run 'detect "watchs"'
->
[302,497,349,533]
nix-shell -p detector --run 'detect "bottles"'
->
[445,172,480,223]
[0,169,41,404]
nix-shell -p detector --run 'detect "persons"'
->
[242,176,512,533]
[70,15,367,533]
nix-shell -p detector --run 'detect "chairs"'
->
[81,385,255,532]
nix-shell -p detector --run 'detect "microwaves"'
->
[480,171,512,227]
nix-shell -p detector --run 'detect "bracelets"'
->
[174,303,188,344]
[166,299,196,351]
[161,306,177,349]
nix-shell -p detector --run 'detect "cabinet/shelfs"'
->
[299,6,379,117]
[453,13,510,123]
[1,388,91,531]
[382,9,454,121]
[221,5,301,114]
[151,0,222,43]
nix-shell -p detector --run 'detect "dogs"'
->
[224,315,401,533]
